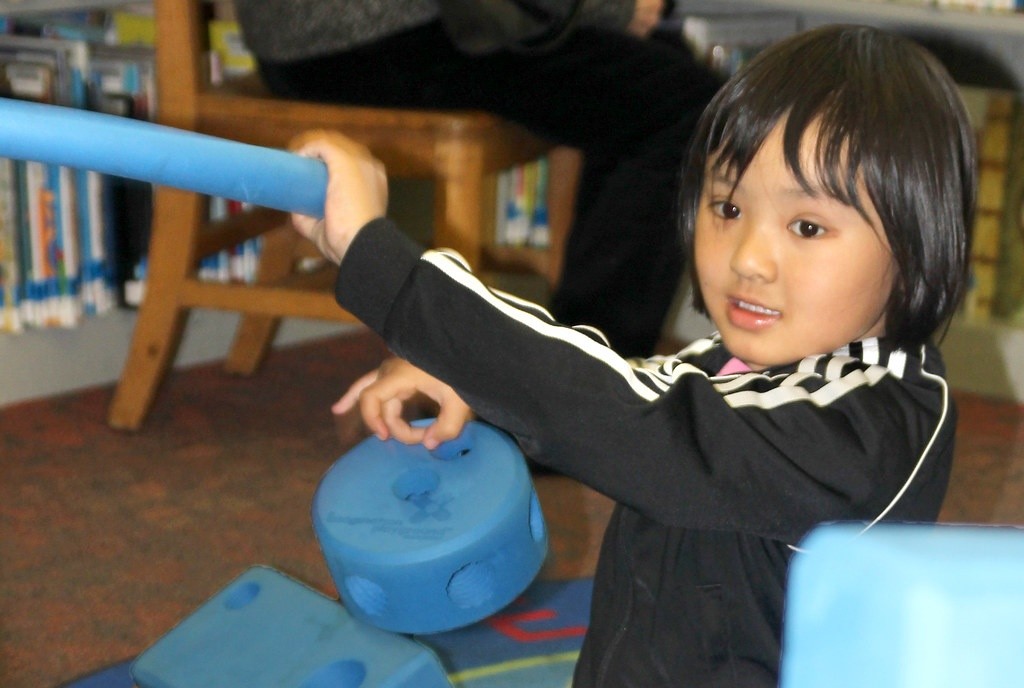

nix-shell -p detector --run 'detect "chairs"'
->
[108,0,584,431]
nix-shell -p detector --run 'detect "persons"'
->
[225,0,716,358]
[287,22,979,688]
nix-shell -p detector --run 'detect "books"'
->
[0,0,261,332]
[496,156,550,247]
